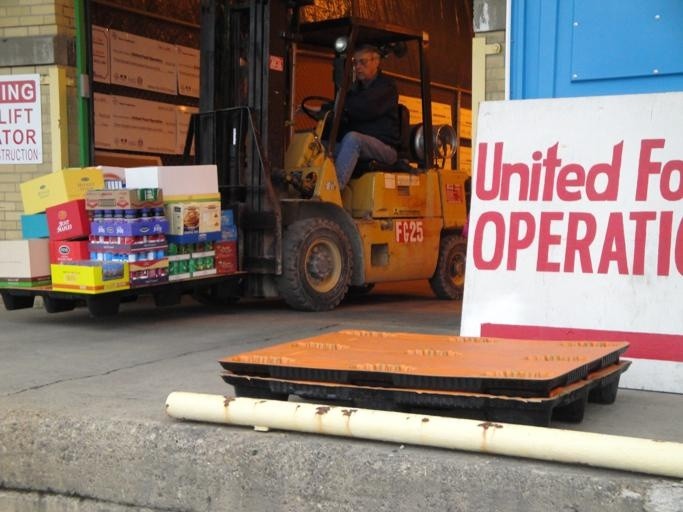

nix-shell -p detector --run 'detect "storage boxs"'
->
[391,91,474,180]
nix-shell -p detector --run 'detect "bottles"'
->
[88,179,214,282]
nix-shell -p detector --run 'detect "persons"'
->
[319,43,400,196]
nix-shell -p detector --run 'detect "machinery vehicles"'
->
[0,2,472,315]
